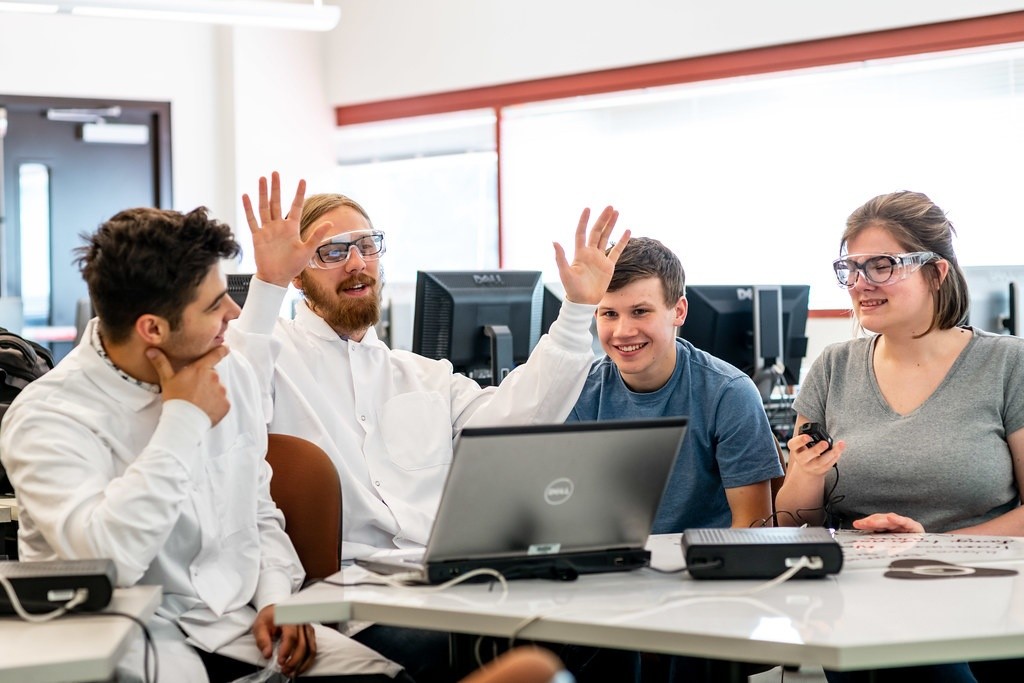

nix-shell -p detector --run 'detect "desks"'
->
[0,328,1024,683]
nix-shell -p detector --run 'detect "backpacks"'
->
[0,327,55,495]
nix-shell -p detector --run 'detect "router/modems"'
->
[680,526,843,581]
[0,559,119,616]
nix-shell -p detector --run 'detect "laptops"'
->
[354,415,691,583]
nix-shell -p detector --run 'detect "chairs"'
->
[263,433,343,630]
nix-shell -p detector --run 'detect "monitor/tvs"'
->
[958,264,1024,340]
[412,269,545,390]
[226,273,254,311]
[677,283,811,401]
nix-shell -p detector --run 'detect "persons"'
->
[775,193,1024,536]
[229,171,632,683]
[563,238,783,529]
[1,209,425,683]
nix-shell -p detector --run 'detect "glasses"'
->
[308,229,386,269]
[832,253,942,290]
